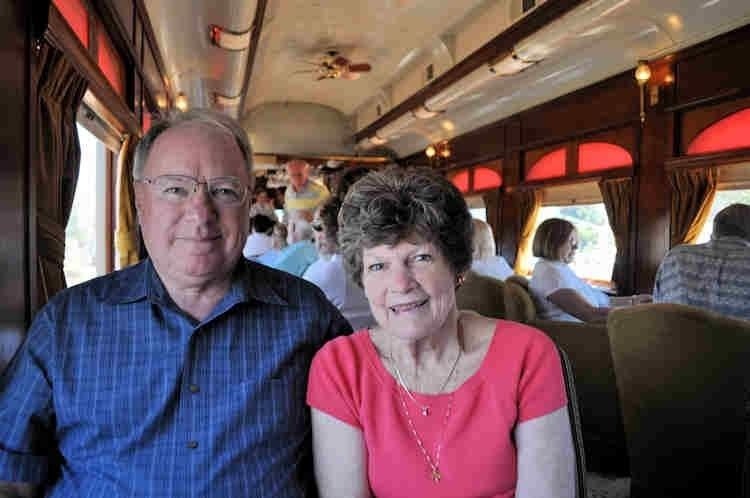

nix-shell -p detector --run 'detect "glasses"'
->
[135,175,250,203]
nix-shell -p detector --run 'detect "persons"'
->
[241,157,516,330]
[653,202,749,321]
[304,157,581,497]
[529,216,621,323]
[0,105,353,497]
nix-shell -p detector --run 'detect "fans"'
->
[292,49,373,83]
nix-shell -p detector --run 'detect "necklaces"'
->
[386,325,467,485]
[390,318,464,418]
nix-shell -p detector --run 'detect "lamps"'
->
[633,57,677,124]
[424,139,452,160]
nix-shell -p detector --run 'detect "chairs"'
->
[535,315,625,477]
[605,305,750,497]
[456,271,614,324]
